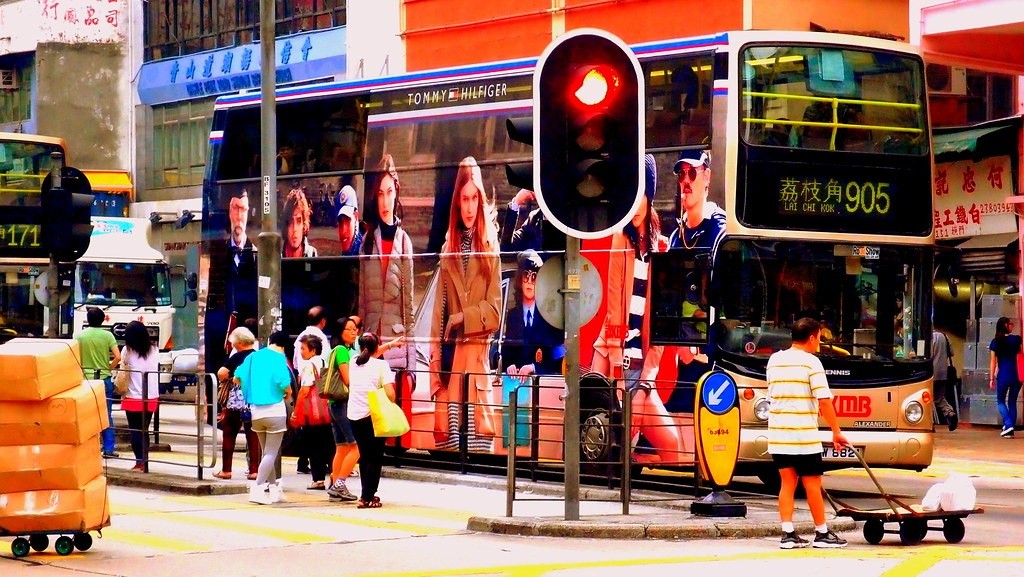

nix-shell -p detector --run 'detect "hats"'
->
[334,184,359,220]
[672,148,711,175]
[645,153,658,206]
[516,248,544,272]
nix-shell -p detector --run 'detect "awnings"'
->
[38,172,132,192]
[934,123,1011,153]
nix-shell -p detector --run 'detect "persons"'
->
[206,147,727,466]
[989,317,1022,439]
[325,318,361,501]
[347,331,403,509]
[894,294,903,346]
[933,331,958,433]
[766,318,847,548]
[277,140,299,174]
[292,306,336,473]
[234,330,292,505]
[121,320,160,472]
[229,318,258,359]
[211,326,259,479]
[802,96,865,150]
[77,307,121,458]
[298,333,332,490]
[350,315,364,349]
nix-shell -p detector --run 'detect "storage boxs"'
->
[961,294,1024,425]
[0,337,110,532]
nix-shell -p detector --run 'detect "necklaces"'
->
[679,195,699,250]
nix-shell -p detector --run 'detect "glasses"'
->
[677,167,704,184]
[344,327,359,333]
[520,270,538,285]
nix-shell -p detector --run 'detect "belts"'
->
[622,354,644,371]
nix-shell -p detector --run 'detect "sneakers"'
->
[812,528,849,548]
[779,530,811,549]
[1000,426,1015,438]
[248,481,287,505]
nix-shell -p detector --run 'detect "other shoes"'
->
[296,466,382,509]
[102,451,145,472]
[947,411,958,431]
[247,473,258,481]
[212,470,232,479]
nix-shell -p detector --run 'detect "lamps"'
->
[149,210,202,229]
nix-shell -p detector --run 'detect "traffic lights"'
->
[41,166,100,263]
[532,30,644,237]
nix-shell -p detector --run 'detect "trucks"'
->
[45,215,198,365]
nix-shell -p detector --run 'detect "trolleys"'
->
[0,520,111,558]
[818,440,986,545]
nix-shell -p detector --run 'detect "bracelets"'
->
[636,382,651,395]
[388,343,391,349]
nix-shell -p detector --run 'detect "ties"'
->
[525,310,533,340]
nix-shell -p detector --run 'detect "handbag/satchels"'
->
[303,361,332,426]
[1016,344,1024,386]
[226,386,245,412]
[366,361,411,438]
[114,347,130,397]
[947,366,958,386]
[316,345,349,402]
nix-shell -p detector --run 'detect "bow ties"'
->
[230,245,243,258]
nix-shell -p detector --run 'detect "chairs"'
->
[646,108,711,146]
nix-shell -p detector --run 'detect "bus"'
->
[0,130,76,346]
[199,28,935,483]
